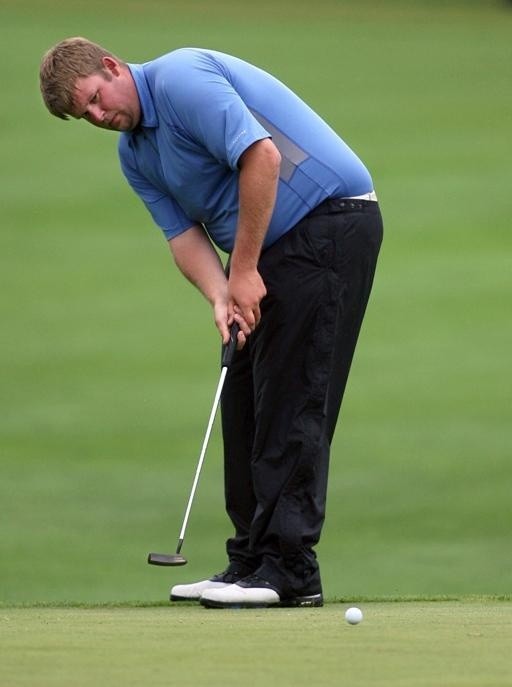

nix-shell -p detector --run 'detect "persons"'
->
[40,37,384,610]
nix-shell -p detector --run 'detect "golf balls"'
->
[344,608,363,626]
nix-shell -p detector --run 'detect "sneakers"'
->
[201,571,326,612]
[170,561,255,600]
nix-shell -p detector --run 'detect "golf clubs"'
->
[148,323,241,566]
